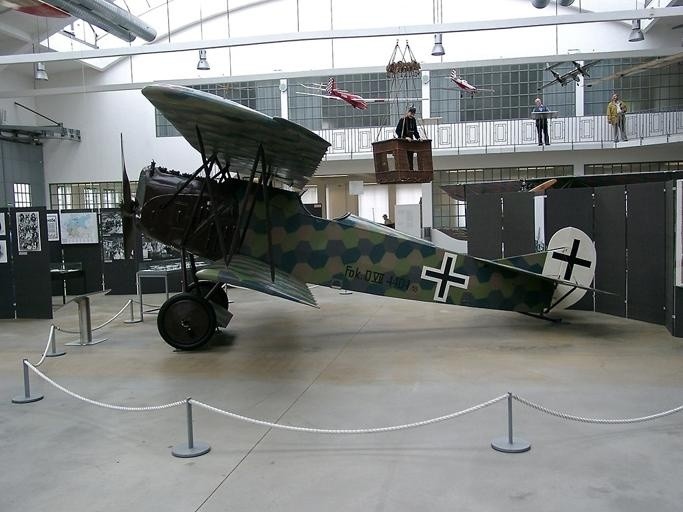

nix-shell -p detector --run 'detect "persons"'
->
[607,94,628,144]
[395,107,421,170]
[533,97,552,145]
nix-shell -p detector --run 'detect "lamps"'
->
[196,50,210,70]
[33,62,48,81]
[432,34,445,56]
[628,20,645,42]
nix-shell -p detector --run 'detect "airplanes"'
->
[293,79,428,111]
[444,71,496,101]
[534,60,599,93]
[114,81,621,354]
